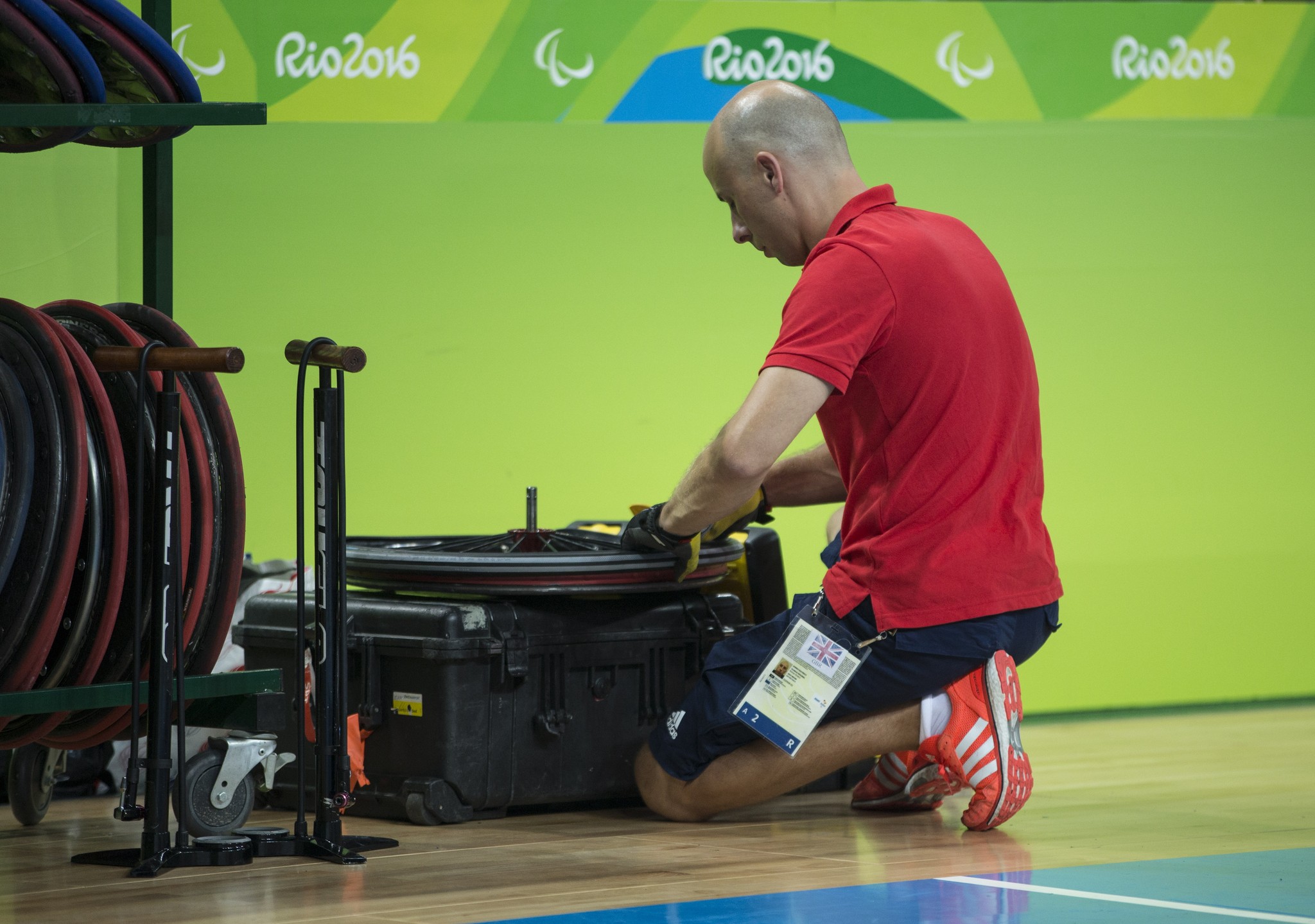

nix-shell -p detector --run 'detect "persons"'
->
[618,78,1065,830]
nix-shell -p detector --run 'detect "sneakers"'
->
[916,651,1033,830]
[852,750,943,812]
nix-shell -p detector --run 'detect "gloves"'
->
[621,501,702,584]
[698,483,776,544]
